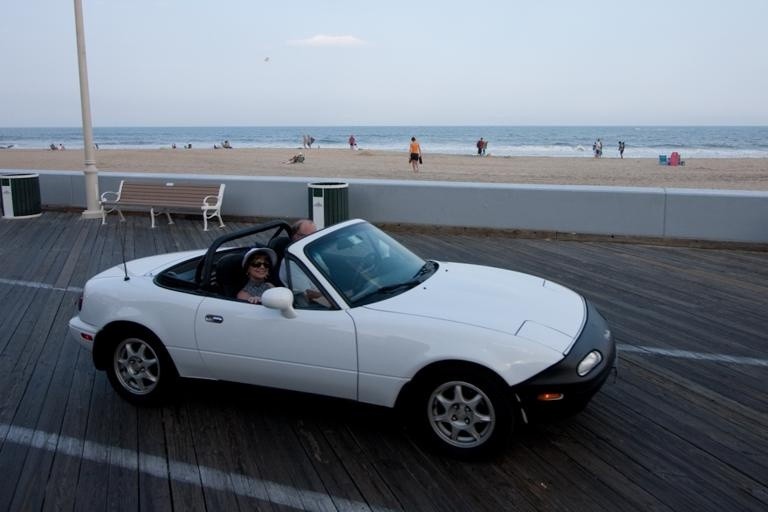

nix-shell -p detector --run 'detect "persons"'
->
[477,137,489,156]
[406,137,423,174]
[279,219,332,308]
[237,247,277,304]
[349,135,358,151]
[214,137,231,149]
[46,142,65,152]
[619,141,626,159]
[171,141,193,151]
[594,139,603,157]
[303,134,312,153]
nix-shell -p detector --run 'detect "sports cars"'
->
[68,217,616,460]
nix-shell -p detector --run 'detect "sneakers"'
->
[419,157,422,164]
[409,159,411,163]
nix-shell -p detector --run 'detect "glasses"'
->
[251,262,271,268]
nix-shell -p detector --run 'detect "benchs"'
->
[98,179,226,232]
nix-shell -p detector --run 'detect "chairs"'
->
[215,235,290,300]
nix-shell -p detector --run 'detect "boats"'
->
[0,143,16,149]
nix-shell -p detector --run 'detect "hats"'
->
[242,247,278,269]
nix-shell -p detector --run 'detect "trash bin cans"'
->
[0,174,42,220]
[660,155,665,166]
[306,181,348,229]
